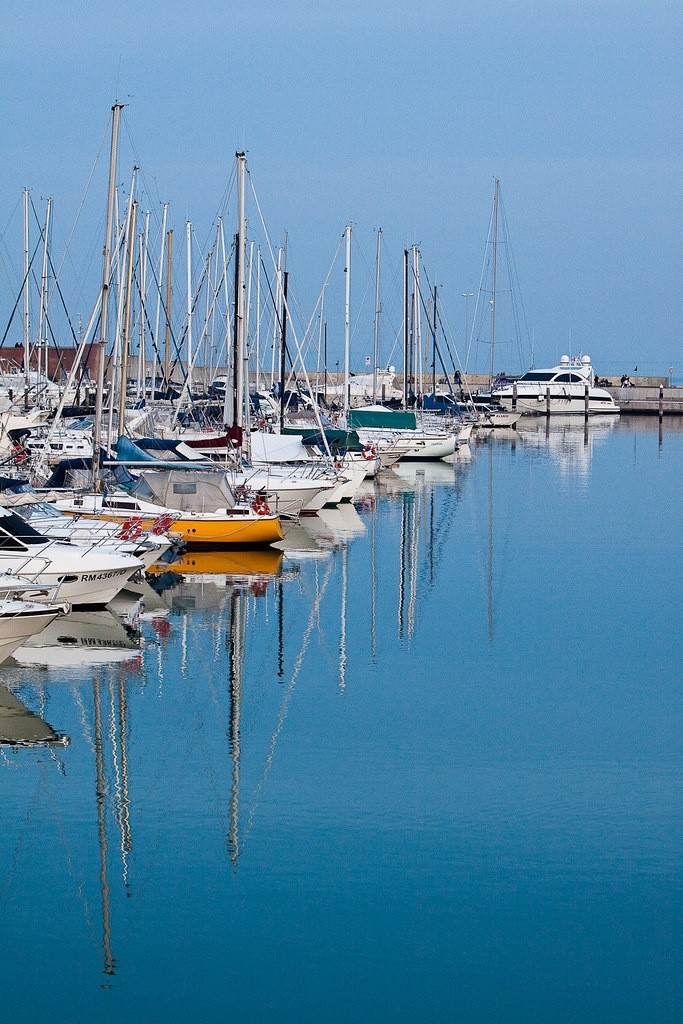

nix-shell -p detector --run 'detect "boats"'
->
[493,355,621,413]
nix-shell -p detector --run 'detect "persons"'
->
[594,373,628,387]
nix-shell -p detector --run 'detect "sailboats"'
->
[0,99,536,664]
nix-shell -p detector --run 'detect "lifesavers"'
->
[362,497,377,512]
[251,580,270,594]
[13,446,27,463]
[152,512,172,535]
[153,616,172,640]
[123,515,143,540]
[249,499,269,513]
[362,445,376,460]
[123,654,144,674]
